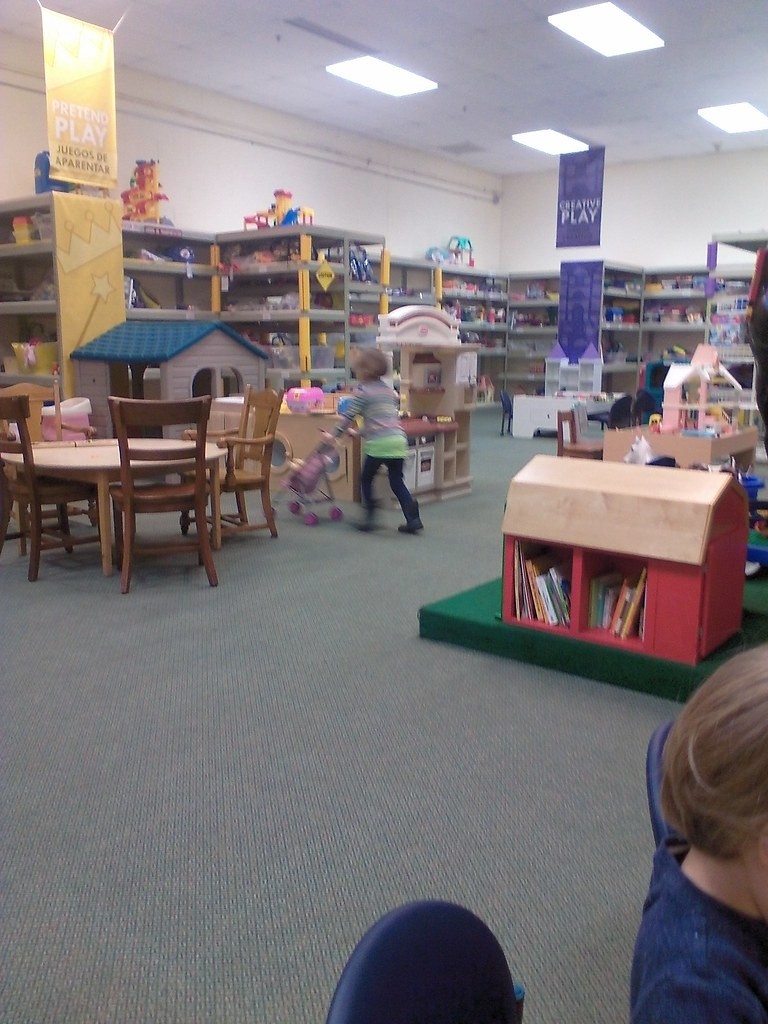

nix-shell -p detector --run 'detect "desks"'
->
[1,437,222,579]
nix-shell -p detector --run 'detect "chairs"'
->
[556,407,604,460]
[110,397,219,593]
[499,390,512,434]
[0,382,99,557]
[328,899,525,1024]
[0,395,100,581]
[177,384,286,550]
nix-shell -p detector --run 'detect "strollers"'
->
[261,417,353,525]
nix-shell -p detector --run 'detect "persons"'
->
[629,642,768,1024]
[745,243,768,456]
[330,348,424,534]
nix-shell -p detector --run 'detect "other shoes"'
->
[348,518,374,533]
[398,516,424,533]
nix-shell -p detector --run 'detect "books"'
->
[514,537,571,629]
[589,566,647,642]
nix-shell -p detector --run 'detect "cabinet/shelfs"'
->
[1,193,711,413]
